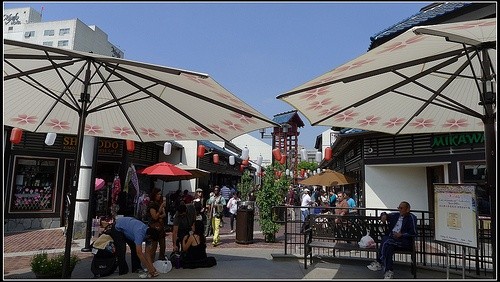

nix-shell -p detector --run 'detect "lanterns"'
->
[272,147,332,179]
[10,128,22,143]
[45,133,56,145]
[127,140,264,177]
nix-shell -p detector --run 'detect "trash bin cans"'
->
[236,209,254,244]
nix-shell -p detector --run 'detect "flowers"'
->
[29,252,79,273]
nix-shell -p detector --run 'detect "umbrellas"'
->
[174,163,210,190]
[2,36,282,276]
[277,18,496,269]
[298,169,361,198]
[135,162,192,198]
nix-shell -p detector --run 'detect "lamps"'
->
[473,164,482,175]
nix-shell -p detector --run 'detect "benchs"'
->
[300,214,419,279]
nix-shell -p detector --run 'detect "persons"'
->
[182,220,207,269]
[111,217,160,276]
[169,182,261,245]
[287,183,356,223]
[172,204,197,252]
[366,202,418,279]
[146,187,167,262]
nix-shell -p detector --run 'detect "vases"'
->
[36,273,71,279]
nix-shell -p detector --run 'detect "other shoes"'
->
[230,230,234,234]
[384,270,395,279]
[212,242,217,245]
[367,260,384,272]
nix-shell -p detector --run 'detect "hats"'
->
[214,185,221,192]
[304,188,310,191]
[195,188,203,191]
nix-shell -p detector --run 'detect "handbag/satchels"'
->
[200,256,216,268]
[169,250,182,268]
[153,257,172,274]
[359,230,377,249]
